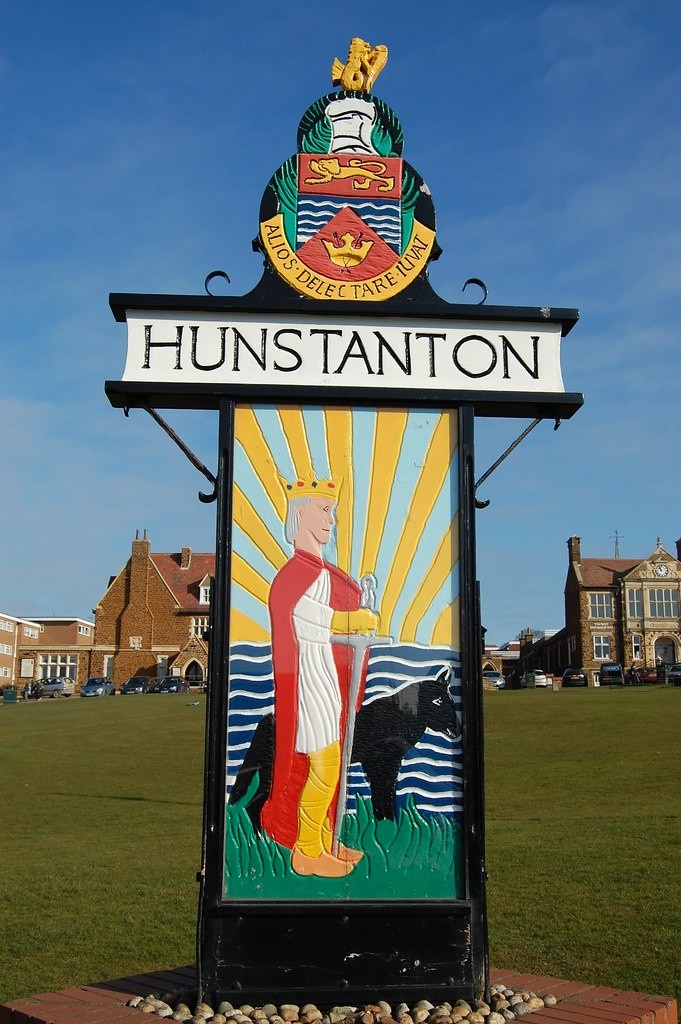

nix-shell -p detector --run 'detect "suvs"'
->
[19,676,76,699]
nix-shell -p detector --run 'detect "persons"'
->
[631,662,638,685]
[22,683,29,703]
[31,680,45,701]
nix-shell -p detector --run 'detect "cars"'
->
[519,668,548,688]
[560,668,589,686]
[636,667,658,684]
[154,676,190,693]
[119,676,157,694]
[481,670,507,689]
[79,677,116,697]
[667,664,681,686]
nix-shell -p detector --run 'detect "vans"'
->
[596,662,625,686]
